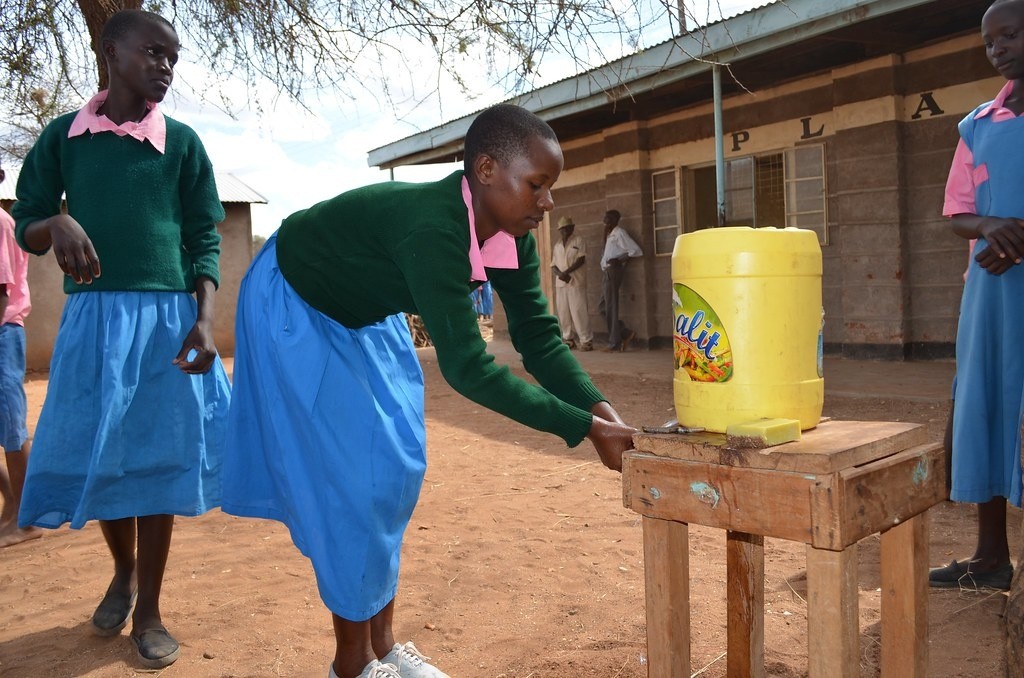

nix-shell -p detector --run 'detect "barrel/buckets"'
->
[671,225,826,435]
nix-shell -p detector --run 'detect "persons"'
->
[598,209,644,353]
[551,214,594,353]
[0,168,45,547]
[221,103,639,678]
[926,1,1024,591]
[12,9,231,664]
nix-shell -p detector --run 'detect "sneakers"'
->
[378,641,451,678]
[329,659,404,678]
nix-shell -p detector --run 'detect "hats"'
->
[557,216,575,229]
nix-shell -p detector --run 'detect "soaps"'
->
[726,417,800,449]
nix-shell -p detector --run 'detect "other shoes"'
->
[623,330,635,352]
[565,339,577,349]
[90,573,138,636]
[130,624,180,667]
[580,340,593,352]
[600,346,621,353]
[929,559,1014,592]
